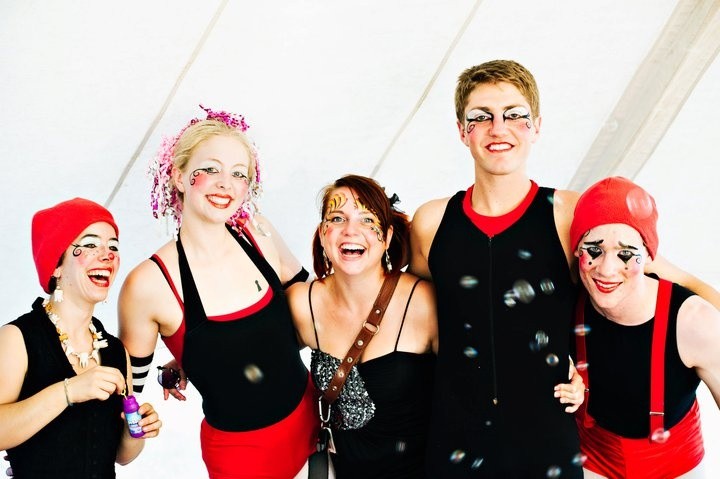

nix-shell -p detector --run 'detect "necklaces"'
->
[42,298,108,369]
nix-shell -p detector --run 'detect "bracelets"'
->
[63,378,74,407]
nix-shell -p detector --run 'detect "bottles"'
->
[122,395,144,438]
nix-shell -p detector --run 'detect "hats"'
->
[31,197,119,295]
[569,177,658,263]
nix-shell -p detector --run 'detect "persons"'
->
[403,58,720,479]
[283,173,586,479]
[567,175,719,478]
[0,196,162,479]
[115,104,315,479]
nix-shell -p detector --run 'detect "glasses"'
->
[157,366,189,391]
